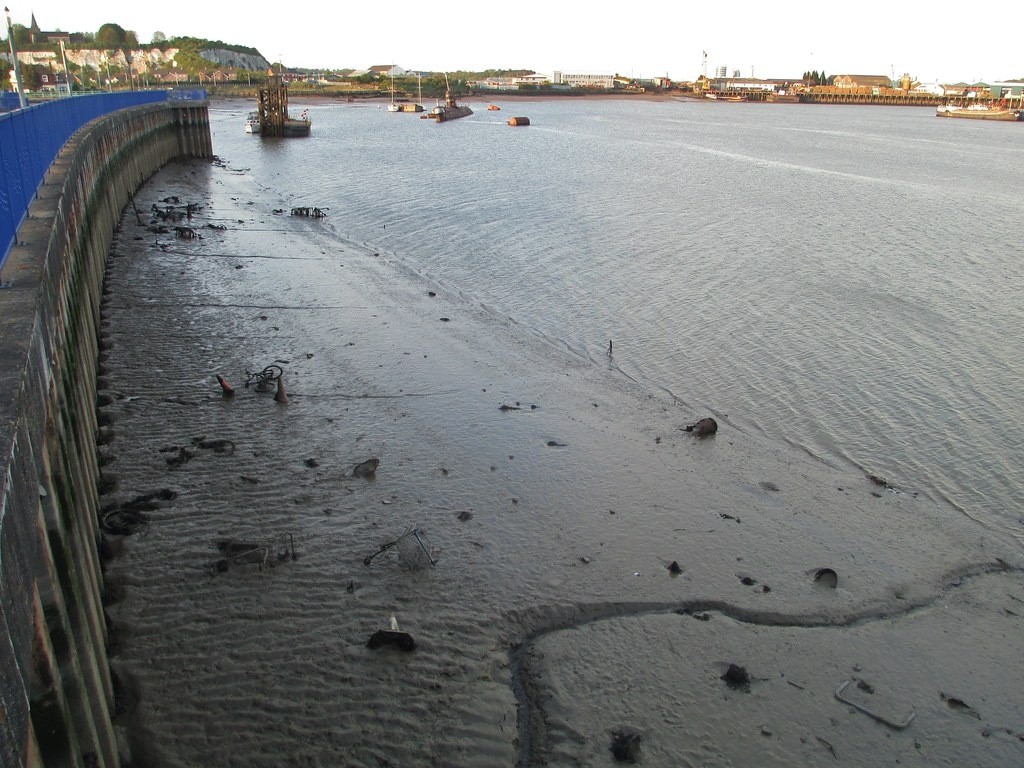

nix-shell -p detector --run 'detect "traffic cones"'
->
[214,374,233,392]
[368,612,418,654]
[274,375,290,403]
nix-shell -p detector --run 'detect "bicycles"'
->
[243,364,284,393]
[160,435,237,470]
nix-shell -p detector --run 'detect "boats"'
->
[427,72,474,123]
[934,104,1021,121]
[244,109,260,133]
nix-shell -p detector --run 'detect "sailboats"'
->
[416,71,427,112]
[385,61,401,113]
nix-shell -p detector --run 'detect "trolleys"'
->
[363,521,439,575]
[204,531,299,578]
